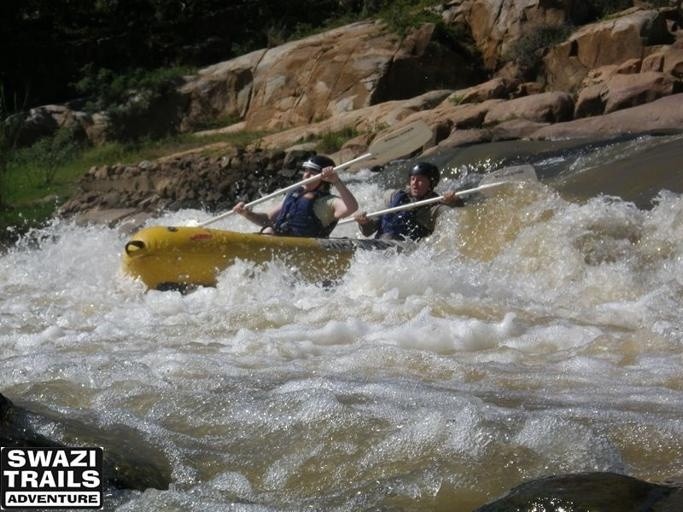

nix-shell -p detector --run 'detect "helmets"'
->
[301,155,336,174]
[407,162,441,186]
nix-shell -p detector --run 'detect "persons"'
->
[230,155,361,239]
[353,162,465,240]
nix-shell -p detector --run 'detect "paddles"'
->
[192,119,433,229]
[337,164,539,223]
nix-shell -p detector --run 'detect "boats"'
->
[121,225,394,290]
